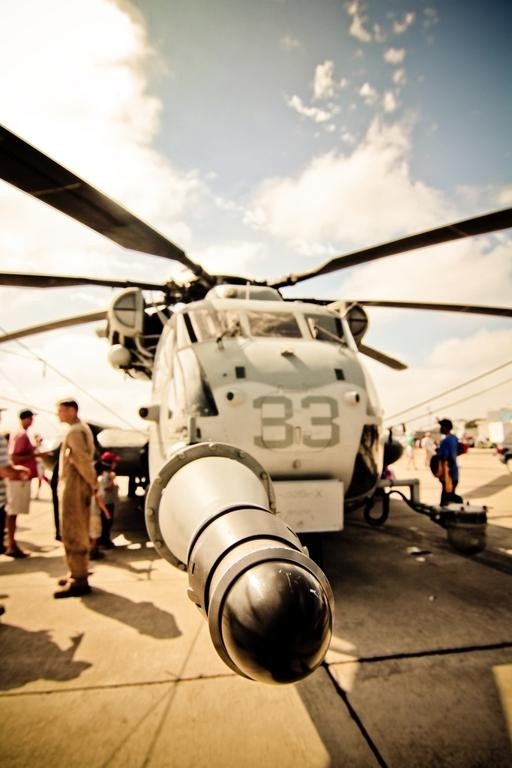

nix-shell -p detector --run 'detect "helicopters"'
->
[0,125,512,687]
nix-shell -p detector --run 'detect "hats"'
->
[100,451,120,461]
[20,411,37,419]
[438,419,453,429]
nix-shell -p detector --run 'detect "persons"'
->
[403,419,495,507]
[1,397,120,614]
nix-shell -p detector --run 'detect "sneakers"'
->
[5,545,29,559]
[88,536,115,561]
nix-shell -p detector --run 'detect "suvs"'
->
[497,432,512,474]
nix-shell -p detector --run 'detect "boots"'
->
[53,576,92,598]
